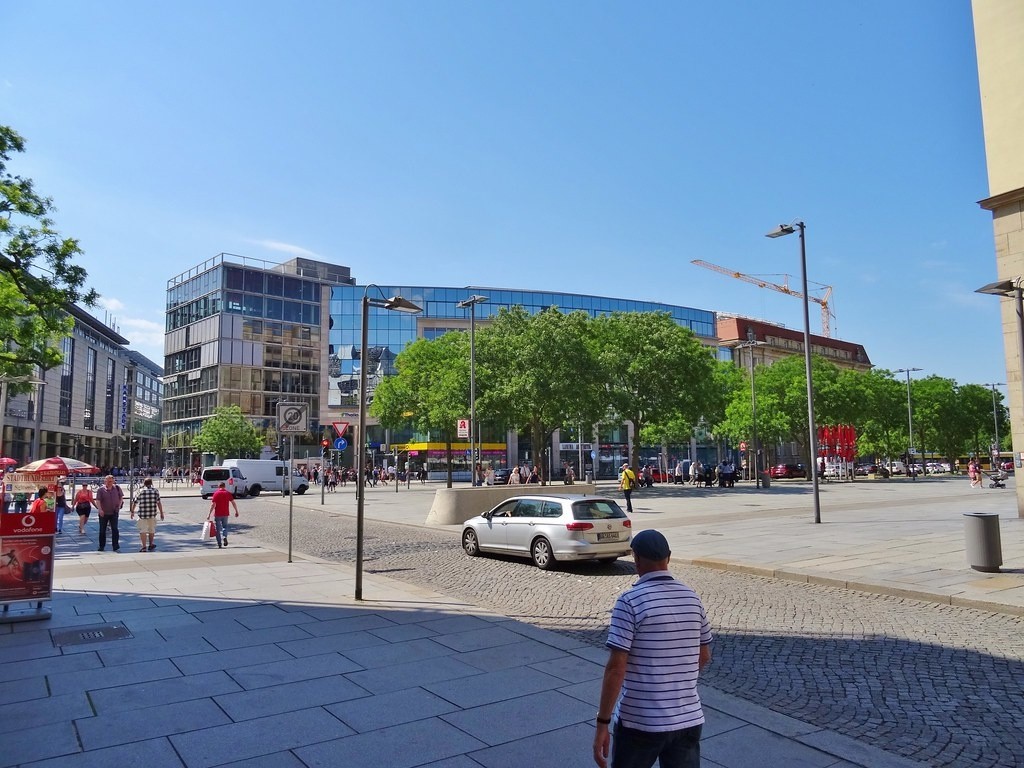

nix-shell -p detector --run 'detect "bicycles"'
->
[90,477,103,492]
[127,477,139,492]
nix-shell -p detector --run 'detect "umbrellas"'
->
[15,455,101,475]
[0,457,18,465]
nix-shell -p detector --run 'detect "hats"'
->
[630,530,669,559]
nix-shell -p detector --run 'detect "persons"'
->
[98,463,202,483]
[130,478,164,552]
[299,461,427,493]
[96,475,123,553]
[505,509,513,517]
[207,482,239,548]
[476,462,498,486]
[56,482,64,535]
[966,455,985,488]
[32,486,54,512]
[618,463,636,513]
[507,461,576,485]
[71,482,98,534]
[592,529,713,768]
[4,467,30,513]
[637,461,735,488]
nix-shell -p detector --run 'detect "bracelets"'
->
[131,511,134,513]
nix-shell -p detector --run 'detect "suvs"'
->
[460,494,634,570]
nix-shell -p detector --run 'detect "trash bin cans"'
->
[585,471,592,484]
[762,473,770,488]
[963,513,1003,573]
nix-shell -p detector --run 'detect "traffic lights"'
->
[338,452,343,459]
[322,438,330,457]
[331,451,335,461]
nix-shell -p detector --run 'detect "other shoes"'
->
[139,547,146,552]
[115,549,121,552]
[218,544,222,548]
[223,538,228,546]
[98,548,104,551]
[971,484,974,488]
[59,530,62,534]
[148,544,156,550]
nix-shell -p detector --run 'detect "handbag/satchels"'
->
[209,521,217,537]
[629,478,638,489]
[77,501,90,511]
[200,520,211,541]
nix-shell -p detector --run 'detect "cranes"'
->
[691,259,835,338]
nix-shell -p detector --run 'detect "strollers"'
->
[981,469,1009,489]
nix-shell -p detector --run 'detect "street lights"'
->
[892,367,923,448]
[974,276,1024,385]
[764,216,822,524]
[733,339,759,489]
[354,283,425,600]
[455,294,491,487]
[982,383,1008,452]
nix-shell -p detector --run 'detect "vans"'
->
[200,466,250,499]
[222,458,310,496]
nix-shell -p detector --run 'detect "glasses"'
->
[83,485,87,486]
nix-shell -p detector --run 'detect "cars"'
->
[493,468,512,484]
[823,460,952,477]
[763,463,807,479]
